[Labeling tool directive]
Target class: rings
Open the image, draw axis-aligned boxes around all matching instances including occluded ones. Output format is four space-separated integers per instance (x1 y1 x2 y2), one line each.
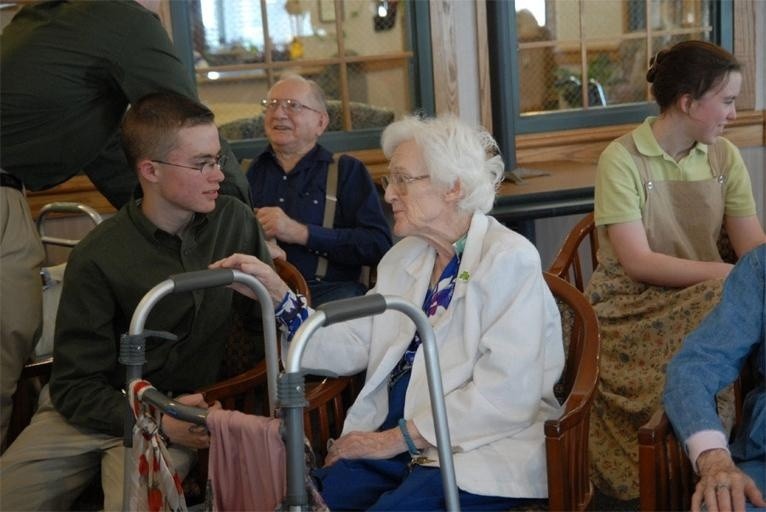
715 484 729 492
337 447 342 454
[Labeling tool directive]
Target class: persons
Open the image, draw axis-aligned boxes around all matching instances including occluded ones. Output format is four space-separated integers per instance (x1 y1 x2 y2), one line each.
244 72 393 310
0 90 277 512
206 111 566 511
579 37 764 512
0 0 257 462
663 240 766 512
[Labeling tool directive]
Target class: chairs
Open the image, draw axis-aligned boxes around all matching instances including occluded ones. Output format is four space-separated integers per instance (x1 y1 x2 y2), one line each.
166 260 312 510
546 213 739 293
304 269 600 512
641 341 758 511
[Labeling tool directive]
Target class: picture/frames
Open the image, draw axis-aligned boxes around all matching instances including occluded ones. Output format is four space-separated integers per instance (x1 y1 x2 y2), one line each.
319 2 347 25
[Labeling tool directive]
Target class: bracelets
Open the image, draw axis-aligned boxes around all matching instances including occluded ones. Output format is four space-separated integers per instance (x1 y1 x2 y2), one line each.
399 417 417 455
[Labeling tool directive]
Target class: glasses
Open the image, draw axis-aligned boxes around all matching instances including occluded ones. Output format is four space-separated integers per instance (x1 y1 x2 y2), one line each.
150 152 228 177
260 97 322 118
379 170 429 196
